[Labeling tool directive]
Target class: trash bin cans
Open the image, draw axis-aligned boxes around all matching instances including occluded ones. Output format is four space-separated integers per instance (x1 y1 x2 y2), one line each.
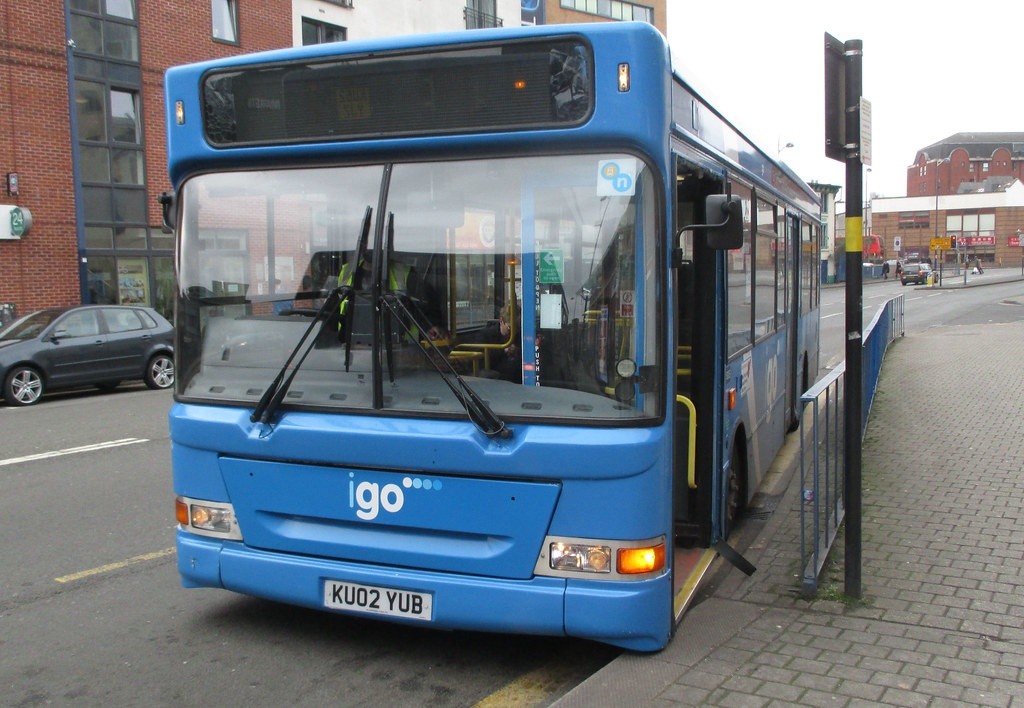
926 272 935 287
934 272 939 283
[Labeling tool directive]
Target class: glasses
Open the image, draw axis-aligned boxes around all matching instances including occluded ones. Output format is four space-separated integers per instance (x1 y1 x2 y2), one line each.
505 323 511 331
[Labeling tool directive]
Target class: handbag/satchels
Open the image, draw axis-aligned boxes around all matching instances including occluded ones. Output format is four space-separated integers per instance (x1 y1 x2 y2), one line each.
973 267 978 273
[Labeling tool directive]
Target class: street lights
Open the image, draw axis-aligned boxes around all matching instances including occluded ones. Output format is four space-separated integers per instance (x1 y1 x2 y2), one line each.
833 200 845 247
865 168 872 237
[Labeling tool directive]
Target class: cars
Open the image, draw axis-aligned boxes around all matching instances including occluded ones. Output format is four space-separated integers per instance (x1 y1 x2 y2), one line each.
0 306 178 408
900 263 939 286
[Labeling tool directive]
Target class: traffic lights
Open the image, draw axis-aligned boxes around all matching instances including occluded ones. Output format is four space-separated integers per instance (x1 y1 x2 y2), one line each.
951 235 957 249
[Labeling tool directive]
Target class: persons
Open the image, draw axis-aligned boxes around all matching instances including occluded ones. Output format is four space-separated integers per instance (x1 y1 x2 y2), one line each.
474 302 522 384
896 259 901 278
882 260 889 279
318 227 447 340
975 258 984 274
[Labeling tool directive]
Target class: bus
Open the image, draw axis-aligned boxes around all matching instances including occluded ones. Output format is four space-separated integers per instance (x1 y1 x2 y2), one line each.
158 22 828 656
835 235 884 260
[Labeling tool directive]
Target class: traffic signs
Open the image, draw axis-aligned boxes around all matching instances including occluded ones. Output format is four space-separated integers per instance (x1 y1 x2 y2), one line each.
539 249 567 284
